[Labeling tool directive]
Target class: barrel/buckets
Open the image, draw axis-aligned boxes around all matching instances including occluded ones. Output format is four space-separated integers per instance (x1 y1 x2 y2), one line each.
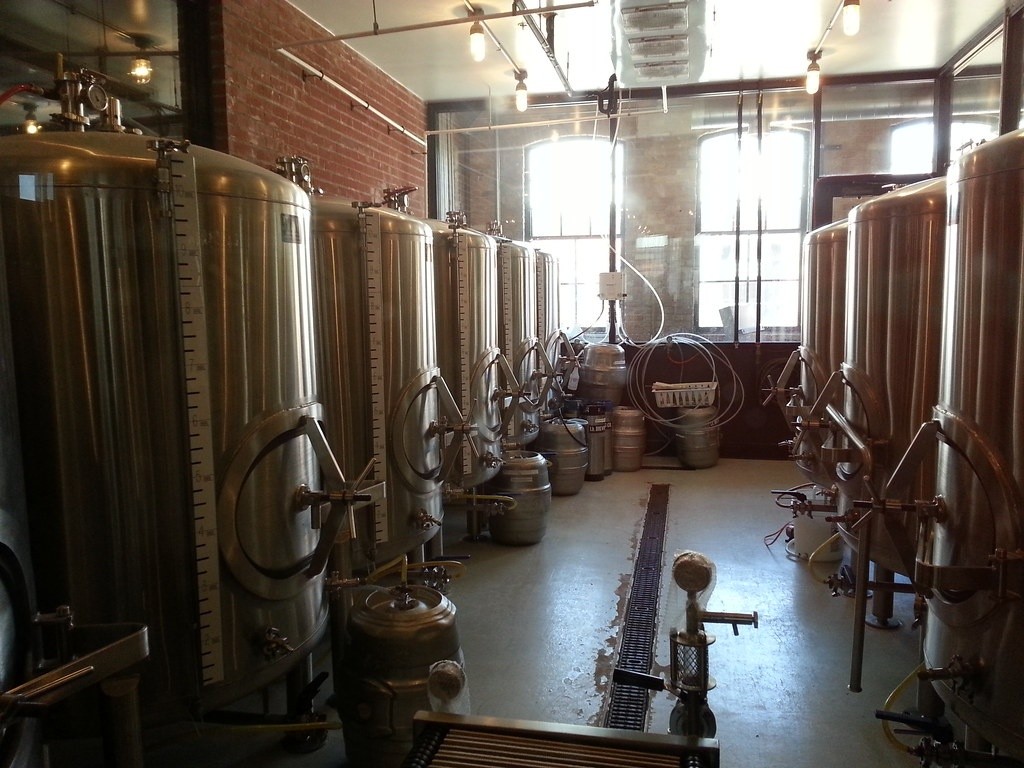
791 487 846 562
339 584 470 768
488 343 646 545
676 406 719 468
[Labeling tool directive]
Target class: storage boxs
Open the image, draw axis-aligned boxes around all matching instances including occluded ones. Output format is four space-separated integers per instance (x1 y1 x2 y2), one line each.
652 381 718 408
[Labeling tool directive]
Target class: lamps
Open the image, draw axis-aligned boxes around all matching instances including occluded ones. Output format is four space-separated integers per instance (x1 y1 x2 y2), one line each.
805 0 863 95
130 40 154 86
461 1 529 113
22 103 38 134
